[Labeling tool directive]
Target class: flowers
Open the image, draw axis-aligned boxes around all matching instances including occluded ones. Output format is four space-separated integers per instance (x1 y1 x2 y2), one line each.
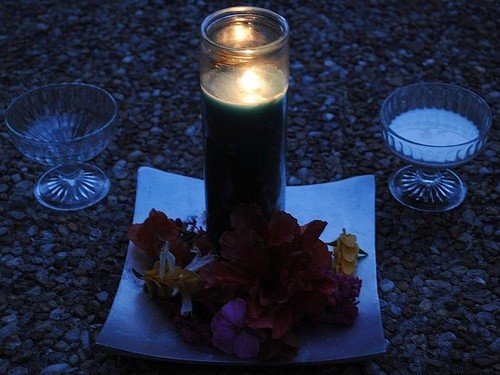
127 210 368 361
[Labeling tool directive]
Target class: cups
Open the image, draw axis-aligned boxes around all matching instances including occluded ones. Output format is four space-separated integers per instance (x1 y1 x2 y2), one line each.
198 7 291 253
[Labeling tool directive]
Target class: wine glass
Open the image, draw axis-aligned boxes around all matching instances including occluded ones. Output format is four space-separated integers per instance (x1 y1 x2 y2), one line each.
4 83 119 212
377 82 493 214
